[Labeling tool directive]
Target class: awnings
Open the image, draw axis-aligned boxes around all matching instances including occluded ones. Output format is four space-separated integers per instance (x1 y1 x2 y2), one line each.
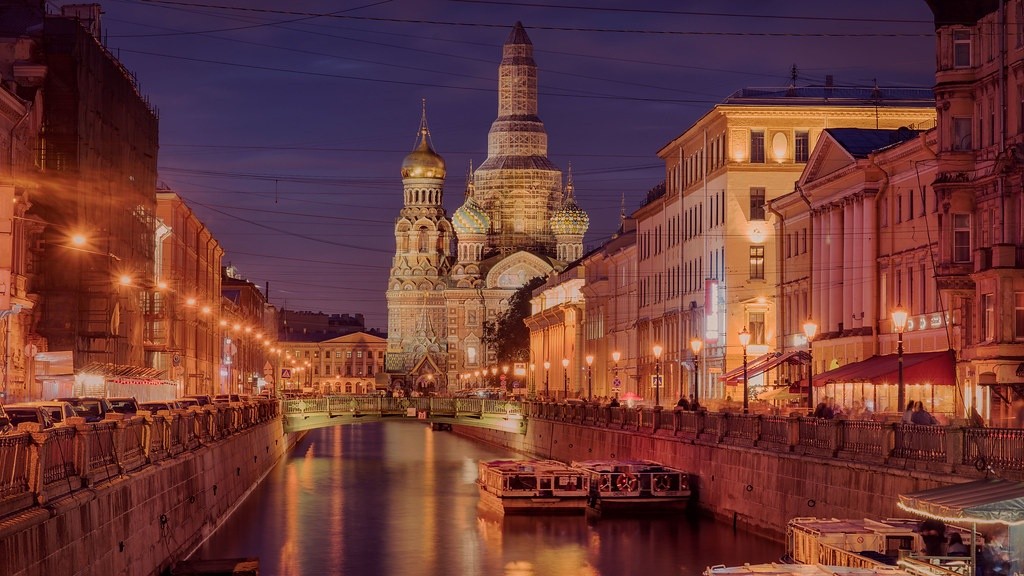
717 350 812 384
789 348 956 394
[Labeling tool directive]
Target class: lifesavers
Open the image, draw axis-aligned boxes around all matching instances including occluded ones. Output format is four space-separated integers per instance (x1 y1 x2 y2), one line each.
616 473 637 491
599 474 613 491
654 475 672 491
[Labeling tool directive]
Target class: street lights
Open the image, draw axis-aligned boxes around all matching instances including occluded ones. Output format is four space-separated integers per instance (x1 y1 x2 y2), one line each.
543 359 552 397
891 303 909 413
802 312 818 417
738 326 752 414
530 362 535 393
502 364 509 392
653 338 663 406
585 350 594 402
465 374 470 390
460 373 463 390
561 355 570 399
611 347 620 400
491 367 498 393
292 367 304 390
474 370 479 388
689 330 702 409
482 368 488 388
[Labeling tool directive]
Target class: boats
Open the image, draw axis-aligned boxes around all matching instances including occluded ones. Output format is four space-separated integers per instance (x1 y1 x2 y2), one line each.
779 478 1024 576
475 458 590 515
570 459 691 512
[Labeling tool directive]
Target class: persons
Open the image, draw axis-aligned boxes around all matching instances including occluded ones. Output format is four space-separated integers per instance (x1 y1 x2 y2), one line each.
911 401 938 426
982 535 1004 576
688 394 700 411
610 399 620 407
828 397 842 420
814 396 833 419
947 533 968 556
611 467 621 491
902 401 914 425
678 395 689 411
920 518 945 557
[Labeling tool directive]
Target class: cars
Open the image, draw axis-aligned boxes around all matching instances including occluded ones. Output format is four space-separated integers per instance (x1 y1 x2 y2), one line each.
0 390 323 432
562 398 584 418
